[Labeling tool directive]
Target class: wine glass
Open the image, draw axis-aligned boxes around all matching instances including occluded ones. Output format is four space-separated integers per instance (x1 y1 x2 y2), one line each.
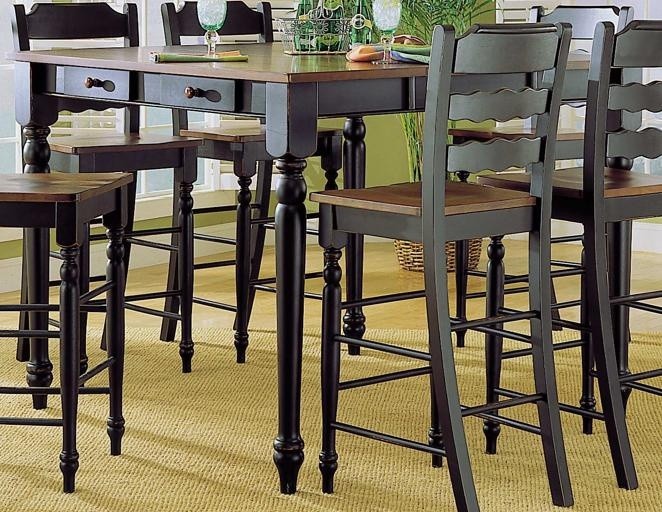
372 0 403 65
198 0 227 58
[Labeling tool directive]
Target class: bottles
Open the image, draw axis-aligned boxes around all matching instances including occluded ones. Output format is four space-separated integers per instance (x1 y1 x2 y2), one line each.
294 0 374 50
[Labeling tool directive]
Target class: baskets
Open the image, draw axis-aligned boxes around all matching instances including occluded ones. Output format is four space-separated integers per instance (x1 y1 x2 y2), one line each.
395 237 482 272
274 15 352 56
